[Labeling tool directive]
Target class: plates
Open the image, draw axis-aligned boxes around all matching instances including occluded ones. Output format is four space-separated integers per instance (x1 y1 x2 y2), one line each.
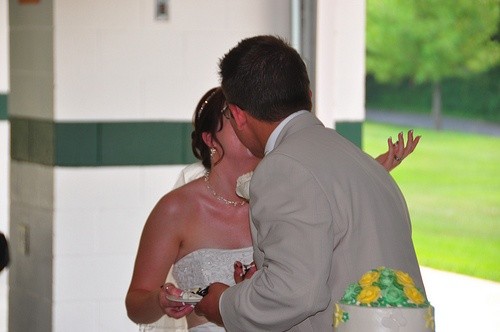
167 298 203 306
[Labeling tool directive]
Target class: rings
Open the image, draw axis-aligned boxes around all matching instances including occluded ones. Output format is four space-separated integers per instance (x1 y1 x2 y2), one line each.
394 156 402 161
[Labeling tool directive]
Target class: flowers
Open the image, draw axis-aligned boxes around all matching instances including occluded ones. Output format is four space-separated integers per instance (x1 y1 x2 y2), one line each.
236 171 254 199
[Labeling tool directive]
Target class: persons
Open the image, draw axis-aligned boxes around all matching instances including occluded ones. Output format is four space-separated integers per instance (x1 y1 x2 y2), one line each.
194 35 428 332
125 86 422 332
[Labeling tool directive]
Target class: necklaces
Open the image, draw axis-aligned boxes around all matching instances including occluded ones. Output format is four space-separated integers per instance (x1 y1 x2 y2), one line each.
203 171 249 208
